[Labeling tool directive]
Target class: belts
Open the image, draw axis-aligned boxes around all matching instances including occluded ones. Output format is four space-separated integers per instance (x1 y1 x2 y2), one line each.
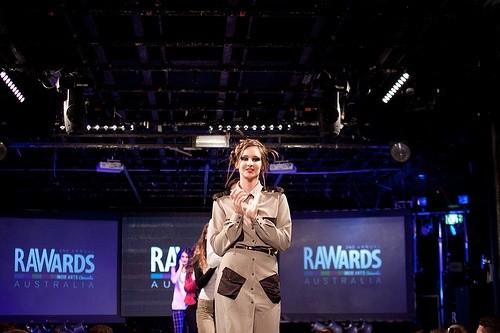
229 244 277 256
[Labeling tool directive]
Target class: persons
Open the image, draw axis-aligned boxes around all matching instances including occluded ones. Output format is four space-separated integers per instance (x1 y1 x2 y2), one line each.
210 140 291 333
170 223 214 333
